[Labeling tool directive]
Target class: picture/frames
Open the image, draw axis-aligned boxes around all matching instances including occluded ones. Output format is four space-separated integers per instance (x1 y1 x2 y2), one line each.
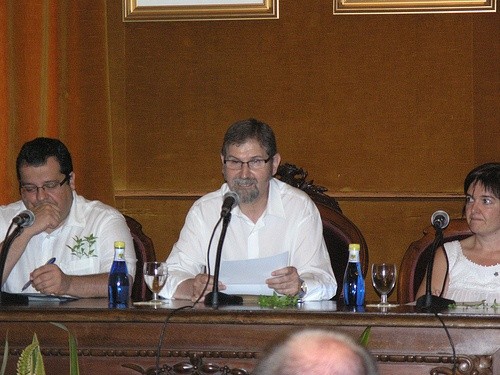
332 0 498 15
121 0 281 22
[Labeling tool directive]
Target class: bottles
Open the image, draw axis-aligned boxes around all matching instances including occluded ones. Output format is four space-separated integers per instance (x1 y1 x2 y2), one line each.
108 240 130 311
343 243 365 306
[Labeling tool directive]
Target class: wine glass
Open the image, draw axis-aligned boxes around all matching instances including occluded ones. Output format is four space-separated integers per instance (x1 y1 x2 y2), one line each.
143 262 168 304
371 263 397 304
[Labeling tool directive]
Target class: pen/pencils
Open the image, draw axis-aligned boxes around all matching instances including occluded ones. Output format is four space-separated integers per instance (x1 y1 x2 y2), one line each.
22 258 56 291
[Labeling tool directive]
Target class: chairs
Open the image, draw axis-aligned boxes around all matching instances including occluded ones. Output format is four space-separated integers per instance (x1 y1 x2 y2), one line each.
398 219 475 306
273 165 370 304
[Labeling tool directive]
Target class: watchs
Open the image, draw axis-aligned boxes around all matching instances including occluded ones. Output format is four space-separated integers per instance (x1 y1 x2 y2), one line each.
299 280 307 298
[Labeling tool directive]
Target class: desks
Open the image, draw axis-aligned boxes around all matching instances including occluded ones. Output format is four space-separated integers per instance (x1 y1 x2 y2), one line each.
0 294 500 375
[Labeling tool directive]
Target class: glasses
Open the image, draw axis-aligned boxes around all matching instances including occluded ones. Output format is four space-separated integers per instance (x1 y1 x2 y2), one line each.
223 153 274 170
18 175 69 195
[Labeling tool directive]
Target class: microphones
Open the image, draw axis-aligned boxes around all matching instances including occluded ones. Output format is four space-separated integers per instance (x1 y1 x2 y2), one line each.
12 210 35 227
219 191 240 216
431 210 450 233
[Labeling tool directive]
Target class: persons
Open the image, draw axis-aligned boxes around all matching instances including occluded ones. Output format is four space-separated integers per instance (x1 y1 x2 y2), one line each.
415 162 500 304
157 118 337 303
252 325 380 375
0 136 136 298
